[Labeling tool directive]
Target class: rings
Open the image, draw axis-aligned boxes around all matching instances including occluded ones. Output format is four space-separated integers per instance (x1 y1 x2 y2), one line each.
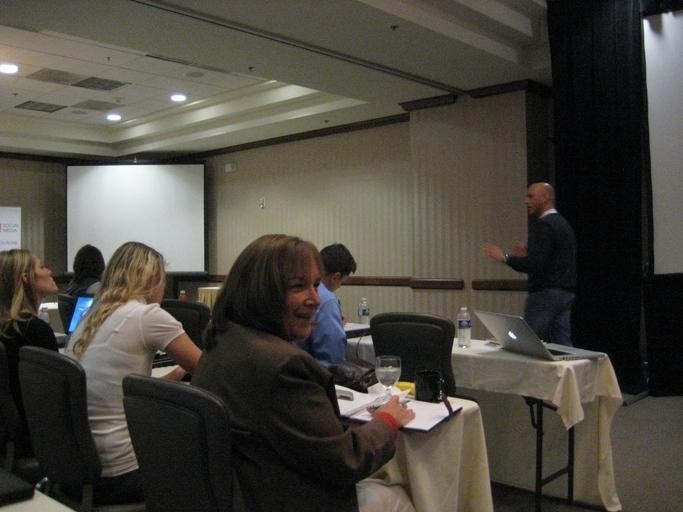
399 402 404 408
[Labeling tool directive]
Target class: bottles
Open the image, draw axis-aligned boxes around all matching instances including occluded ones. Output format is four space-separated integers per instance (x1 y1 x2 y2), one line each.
358 297 369 325
457 306 471 349
39 307 51 327
178 290 189 301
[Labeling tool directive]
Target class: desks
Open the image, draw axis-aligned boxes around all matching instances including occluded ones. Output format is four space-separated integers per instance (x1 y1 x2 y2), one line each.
345 314 624 511
198 285 222 313
330 384 495 512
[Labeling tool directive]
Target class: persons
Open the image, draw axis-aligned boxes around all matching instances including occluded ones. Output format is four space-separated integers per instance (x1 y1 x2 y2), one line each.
66 244 105 298
0 250 59 457
480 182 578 346
293 244 357 366
64 241 202 505
189 235 415 512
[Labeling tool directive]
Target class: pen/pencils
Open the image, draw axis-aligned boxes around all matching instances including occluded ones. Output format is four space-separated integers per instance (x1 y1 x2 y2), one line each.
366 399 410 413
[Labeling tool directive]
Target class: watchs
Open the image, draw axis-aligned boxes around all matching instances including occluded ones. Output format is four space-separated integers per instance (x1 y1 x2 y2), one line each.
503 249 512 265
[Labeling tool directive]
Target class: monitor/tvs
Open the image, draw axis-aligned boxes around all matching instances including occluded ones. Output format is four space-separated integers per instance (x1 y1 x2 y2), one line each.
65 294 95 336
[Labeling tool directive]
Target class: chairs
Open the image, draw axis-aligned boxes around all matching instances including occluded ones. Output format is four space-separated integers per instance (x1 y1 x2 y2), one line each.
57 294 78 334
370 312 456 402
122 374 243 512
160 300 210 345
18 345 102 510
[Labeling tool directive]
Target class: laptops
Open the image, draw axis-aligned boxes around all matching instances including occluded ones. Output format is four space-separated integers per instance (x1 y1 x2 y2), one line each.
473 308 604 362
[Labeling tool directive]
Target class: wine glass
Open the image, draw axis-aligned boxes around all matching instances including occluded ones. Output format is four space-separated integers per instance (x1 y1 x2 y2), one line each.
376 353 401 395
